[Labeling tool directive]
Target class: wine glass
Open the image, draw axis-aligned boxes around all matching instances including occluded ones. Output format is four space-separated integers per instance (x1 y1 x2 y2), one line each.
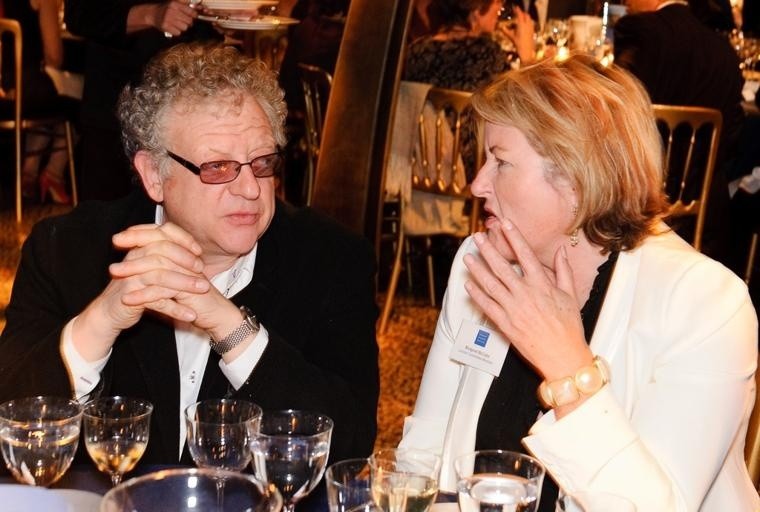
83 396 153 487
500 5 760 80
250 408 334 511
184 399 262 512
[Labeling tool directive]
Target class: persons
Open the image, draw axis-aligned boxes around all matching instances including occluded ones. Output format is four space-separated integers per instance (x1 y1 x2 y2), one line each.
1 43 380 512
612 0 745 240
1 0 70 205
63 0 247 190
402 1 536 221
398 55 758 512
500 0 599 42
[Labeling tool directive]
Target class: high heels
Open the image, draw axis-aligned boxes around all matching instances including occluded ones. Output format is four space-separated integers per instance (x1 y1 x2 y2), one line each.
40 172 69 205
21 173 36 202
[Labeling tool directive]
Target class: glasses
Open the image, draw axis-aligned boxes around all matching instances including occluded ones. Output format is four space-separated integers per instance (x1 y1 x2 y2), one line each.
164 147 285 185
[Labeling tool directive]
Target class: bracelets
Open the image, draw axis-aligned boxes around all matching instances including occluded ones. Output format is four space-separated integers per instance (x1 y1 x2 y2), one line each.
536 355 611 404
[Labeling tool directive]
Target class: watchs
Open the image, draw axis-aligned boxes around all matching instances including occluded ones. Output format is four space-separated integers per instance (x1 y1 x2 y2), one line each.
207 304 261 356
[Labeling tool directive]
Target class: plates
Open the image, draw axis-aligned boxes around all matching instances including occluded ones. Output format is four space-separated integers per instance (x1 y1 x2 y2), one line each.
195 12 299 31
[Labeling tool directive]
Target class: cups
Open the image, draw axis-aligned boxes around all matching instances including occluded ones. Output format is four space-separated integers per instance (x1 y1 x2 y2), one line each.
1 396 83 486
327 458 406 510
101 467 284 511
368 448 443 511
454 451 544 511
